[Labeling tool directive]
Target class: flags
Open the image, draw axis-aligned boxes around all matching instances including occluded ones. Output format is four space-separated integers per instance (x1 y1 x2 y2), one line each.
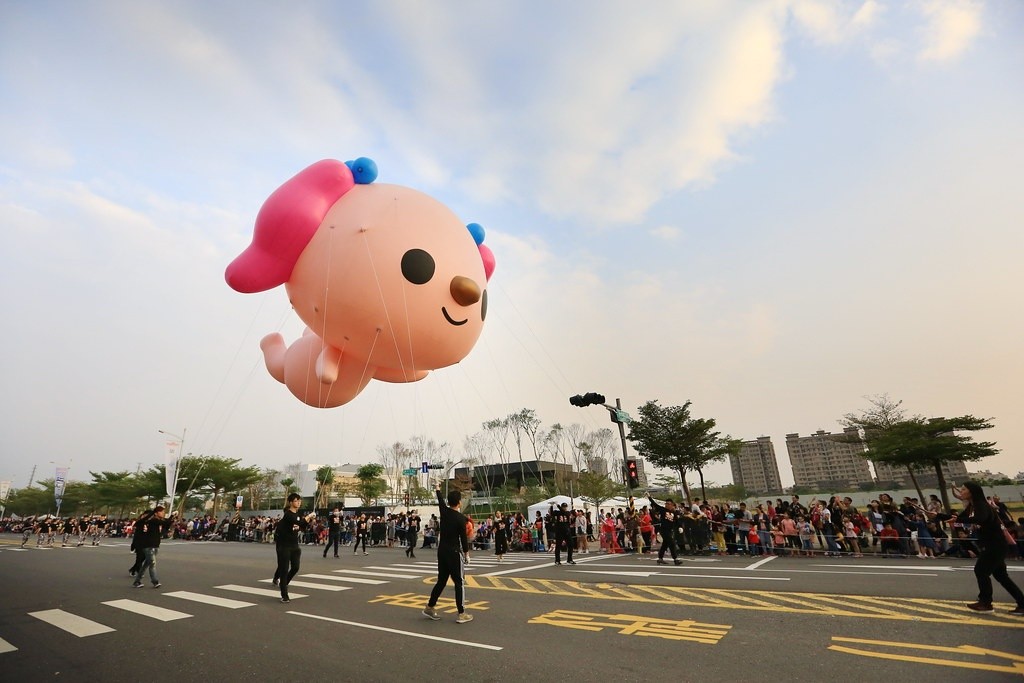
165 441 178 497
56 468 67 507
0 481 11 511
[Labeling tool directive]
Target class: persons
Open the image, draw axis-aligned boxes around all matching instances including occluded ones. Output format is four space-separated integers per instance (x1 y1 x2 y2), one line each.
133 507 178 588
422 477 473 623
322 507 343 558
273 493 316 603
956 481 1024 615
405 510 421 558
128 510 154 578
0 511 440 549
550 502 576 566
644 492 683 565
354 513 370 556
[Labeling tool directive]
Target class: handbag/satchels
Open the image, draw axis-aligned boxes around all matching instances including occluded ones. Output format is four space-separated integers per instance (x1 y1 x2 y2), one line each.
1001 524 1018 546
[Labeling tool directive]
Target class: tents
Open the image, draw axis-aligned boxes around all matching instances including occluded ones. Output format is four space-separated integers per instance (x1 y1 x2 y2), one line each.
528 495 595 532
599 496 665 519
465 484 1024 561
577 496 599 525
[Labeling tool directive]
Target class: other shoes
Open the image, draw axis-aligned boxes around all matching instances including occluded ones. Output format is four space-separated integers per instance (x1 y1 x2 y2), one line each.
323 551 326 557
555 561 561 566
132 581 144 588
282 597 290 603
405 550 409 557
674 560 683 565
273 579 280 586
567 560 577 564
364 552 369 556
677 552 972 560
354 551 358 556
577 549 616 555
497 556 504 562
657 559 669 565
129 570 137 577
334 554 339 557
154 583 163 589
411 554 416 558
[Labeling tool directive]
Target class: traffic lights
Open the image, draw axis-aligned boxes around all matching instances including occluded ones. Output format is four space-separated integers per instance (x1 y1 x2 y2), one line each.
570 394 585 408
584 392 605 405
627 459 640 488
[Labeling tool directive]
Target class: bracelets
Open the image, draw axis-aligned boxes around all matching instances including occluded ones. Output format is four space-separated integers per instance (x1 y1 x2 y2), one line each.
956 488 958 490
742 520 746 522
435 484 439 486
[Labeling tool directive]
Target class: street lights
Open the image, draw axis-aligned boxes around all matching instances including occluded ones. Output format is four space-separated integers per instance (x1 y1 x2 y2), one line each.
158 429 184 517
50 460 71 516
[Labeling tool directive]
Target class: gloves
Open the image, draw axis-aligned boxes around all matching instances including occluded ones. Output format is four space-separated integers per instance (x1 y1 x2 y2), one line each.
464 555 471 566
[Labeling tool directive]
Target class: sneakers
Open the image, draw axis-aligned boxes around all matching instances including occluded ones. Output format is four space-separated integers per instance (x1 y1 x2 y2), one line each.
966 601 995 613
456 613 474 623
422 608 441 621
1008 606 1024 616
